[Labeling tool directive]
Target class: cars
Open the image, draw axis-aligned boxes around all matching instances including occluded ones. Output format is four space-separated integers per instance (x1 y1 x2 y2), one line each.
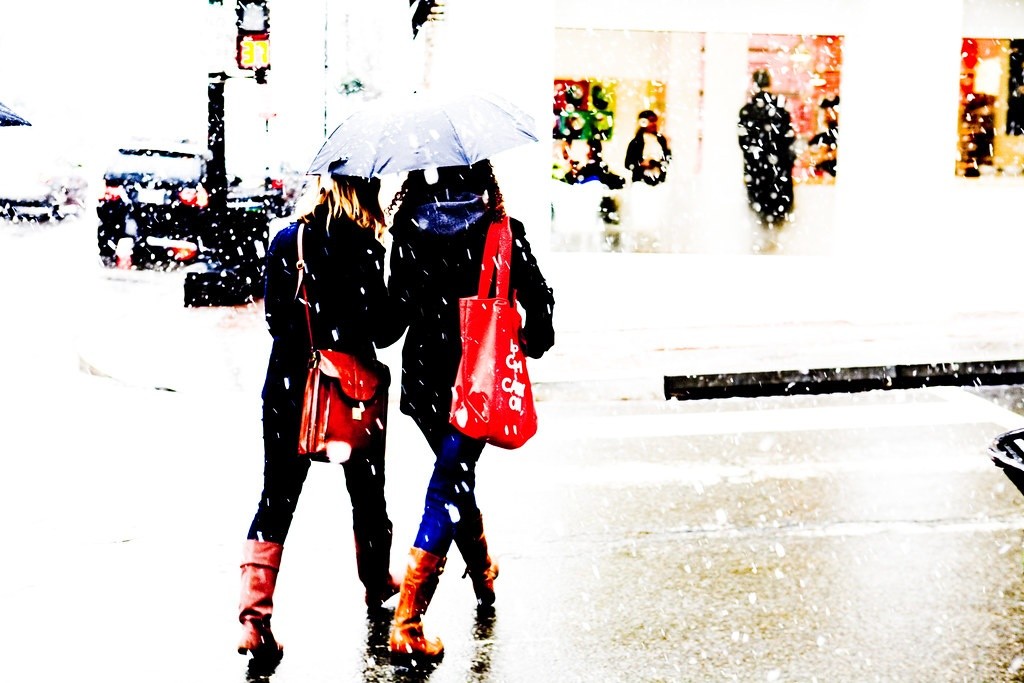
94 135 304 273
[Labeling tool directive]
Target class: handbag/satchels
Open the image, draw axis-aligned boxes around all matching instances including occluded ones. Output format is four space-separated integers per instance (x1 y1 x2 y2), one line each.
447 217 538 449
295 349 391 465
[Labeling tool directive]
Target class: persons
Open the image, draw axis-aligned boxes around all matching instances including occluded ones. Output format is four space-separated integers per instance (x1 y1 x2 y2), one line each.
802 94 841 185
959 71 996 178
625 110 671 187
737 68 797 253
555 132 627 247
384 158 556 656
234 172 403 660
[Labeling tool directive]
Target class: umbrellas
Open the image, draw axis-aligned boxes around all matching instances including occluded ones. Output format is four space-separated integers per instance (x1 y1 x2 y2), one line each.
305 86 540 184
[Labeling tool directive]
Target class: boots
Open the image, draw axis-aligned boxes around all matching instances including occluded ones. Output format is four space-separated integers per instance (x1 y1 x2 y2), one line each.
386 547 448 667
235 538 284 660
354 518 405 617
453 513 499 606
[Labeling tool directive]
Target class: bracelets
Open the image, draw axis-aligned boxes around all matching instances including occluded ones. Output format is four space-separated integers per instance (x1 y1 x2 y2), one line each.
827 151 833 159
